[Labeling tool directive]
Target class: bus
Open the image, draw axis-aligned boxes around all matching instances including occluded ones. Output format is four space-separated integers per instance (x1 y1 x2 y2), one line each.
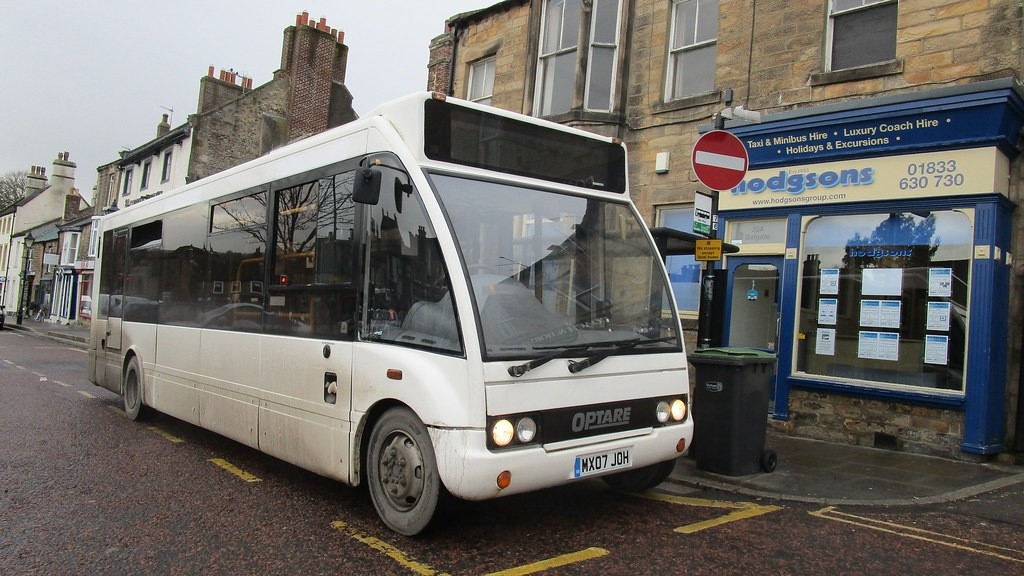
85 90 696 542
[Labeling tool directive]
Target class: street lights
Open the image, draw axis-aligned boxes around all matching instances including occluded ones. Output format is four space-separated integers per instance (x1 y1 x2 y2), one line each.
15 230 35 327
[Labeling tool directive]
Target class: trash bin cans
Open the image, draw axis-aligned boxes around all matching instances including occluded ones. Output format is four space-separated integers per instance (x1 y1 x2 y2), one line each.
686 346 777 476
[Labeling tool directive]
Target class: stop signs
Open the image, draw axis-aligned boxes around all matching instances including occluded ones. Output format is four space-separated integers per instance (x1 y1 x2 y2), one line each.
691 129 749 192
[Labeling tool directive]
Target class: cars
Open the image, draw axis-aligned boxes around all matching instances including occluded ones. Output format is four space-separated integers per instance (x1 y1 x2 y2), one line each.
99 293 315 338
80 295 92 319
